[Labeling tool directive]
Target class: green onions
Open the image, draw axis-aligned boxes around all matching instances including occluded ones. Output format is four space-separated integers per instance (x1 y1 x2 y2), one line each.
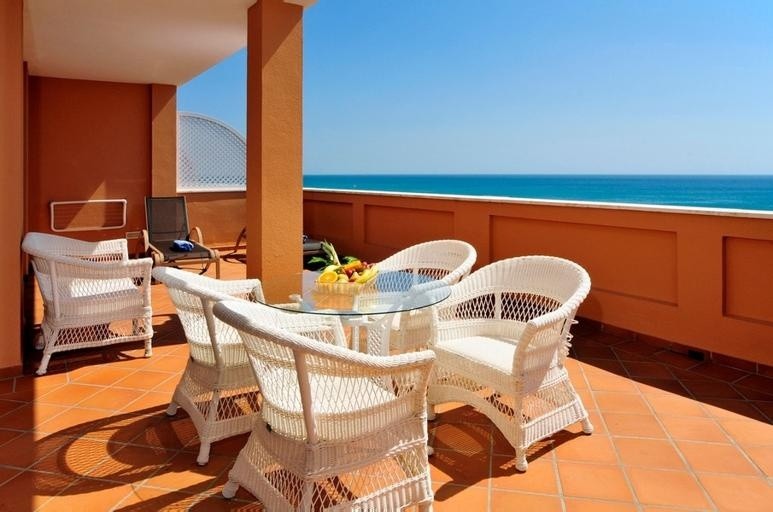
307 240 341 265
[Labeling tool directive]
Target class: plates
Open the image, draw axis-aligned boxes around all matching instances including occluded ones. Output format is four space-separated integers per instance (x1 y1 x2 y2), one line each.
313 271 379 296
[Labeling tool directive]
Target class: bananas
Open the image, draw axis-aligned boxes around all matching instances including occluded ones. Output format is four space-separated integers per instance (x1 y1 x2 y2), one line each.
353 268 376 283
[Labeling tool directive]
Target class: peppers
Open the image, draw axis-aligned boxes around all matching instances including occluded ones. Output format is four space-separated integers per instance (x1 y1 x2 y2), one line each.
342 256 358 264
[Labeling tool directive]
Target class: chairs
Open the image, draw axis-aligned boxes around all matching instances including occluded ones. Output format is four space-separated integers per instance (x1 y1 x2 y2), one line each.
352 239 478 350
21 232 155 377
233 225 325 269
422 254 594 473
151 266 265 468
141 195 221 284
213 297 434 512
